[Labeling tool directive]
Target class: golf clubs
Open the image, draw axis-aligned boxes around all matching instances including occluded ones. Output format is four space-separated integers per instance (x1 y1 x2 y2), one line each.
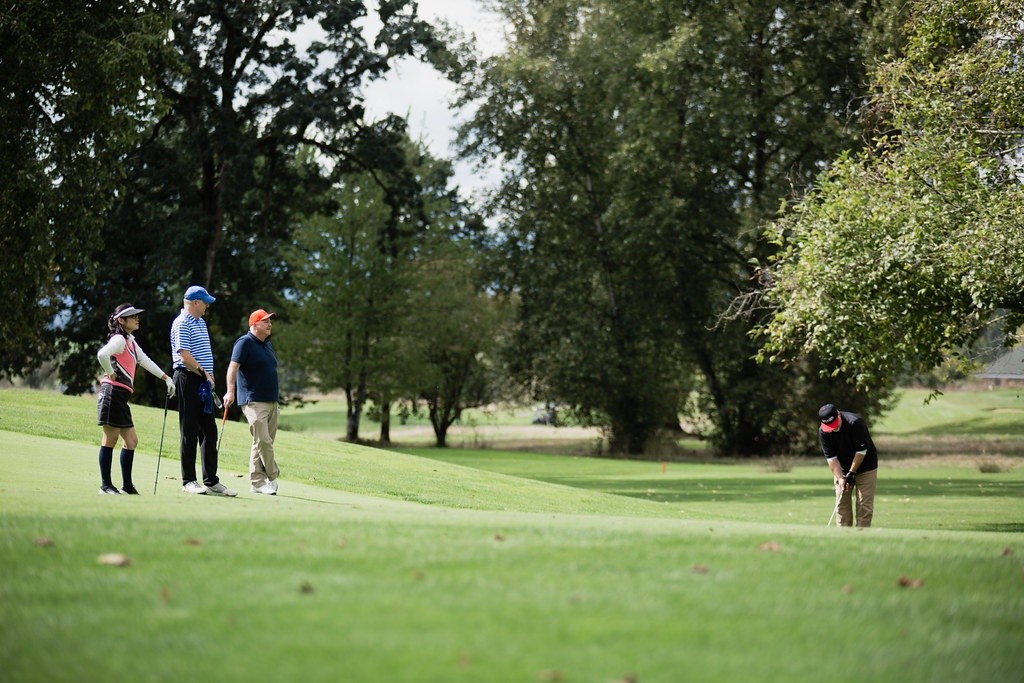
826 490 844 528
217 403 230 454
153 386 173 496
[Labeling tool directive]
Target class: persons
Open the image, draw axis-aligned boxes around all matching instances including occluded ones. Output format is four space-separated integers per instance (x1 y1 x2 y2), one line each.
223 309 281 496
170 286 239 497
97 304 176 496
819 404 878 527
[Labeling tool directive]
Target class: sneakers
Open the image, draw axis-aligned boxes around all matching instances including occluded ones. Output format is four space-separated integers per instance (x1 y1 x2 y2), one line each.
121 486 139 495
183 480 207 494
209 482 238 496
99 485 123 495
250 485 277 495
268 480 277 490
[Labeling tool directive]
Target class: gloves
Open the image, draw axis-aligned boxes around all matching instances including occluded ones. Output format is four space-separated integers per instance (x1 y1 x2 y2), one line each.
165 377 175 399
844 470 857 490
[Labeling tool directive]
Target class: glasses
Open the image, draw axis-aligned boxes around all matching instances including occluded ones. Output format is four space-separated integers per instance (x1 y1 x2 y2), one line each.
127 315 138 318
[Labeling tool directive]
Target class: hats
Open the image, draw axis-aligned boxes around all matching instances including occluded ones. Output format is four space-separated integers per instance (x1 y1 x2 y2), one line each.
819 404 839 432
249 309 277 326
113 307 144 320
184 286 216 303
